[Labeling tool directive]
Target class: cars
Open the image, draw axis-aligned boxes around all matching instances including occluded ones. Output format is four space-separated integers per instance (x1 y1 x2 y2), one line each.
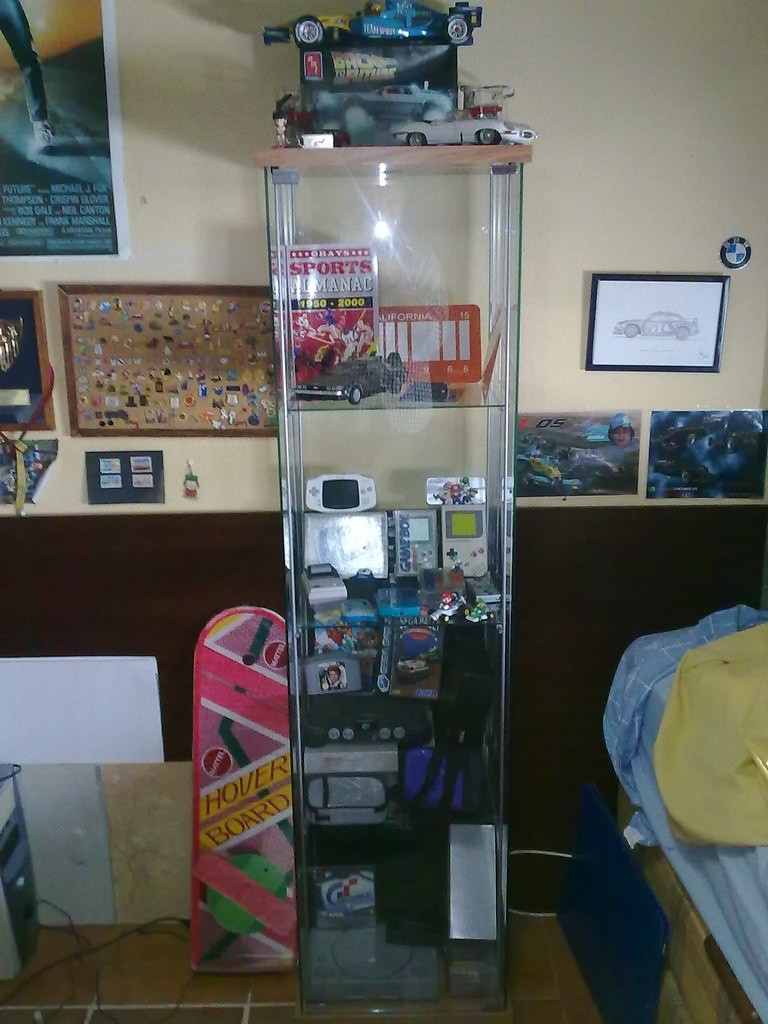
393 114 538 144
312 84 457 122
296 357 391 406
319 128 350 146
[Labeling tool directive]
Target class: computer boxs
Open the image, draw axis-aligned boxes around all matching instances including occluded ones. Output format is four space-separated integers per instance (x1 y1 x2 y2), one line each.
0 764 41 981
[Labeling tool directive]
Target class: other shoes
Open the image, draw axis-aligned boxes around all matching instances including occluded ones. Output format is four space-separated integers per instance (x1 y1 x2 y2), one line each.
32 121 53 152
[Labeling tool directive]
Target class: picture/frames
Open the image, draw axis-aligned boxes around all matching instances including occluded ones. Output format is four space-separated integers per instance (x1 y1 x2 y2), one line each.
585 273 731 373
0 289 56 431
56 283 278 436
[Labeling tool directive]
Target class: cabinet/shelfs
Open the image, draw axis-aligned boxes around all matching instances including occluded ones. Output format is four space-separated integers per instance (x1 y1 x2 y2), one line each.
256 147 532 1016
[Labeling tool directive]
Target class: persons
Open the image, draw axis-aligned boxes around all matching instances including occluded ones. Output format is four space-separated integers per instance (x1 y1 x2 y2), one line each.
604 413 641 468
327 665 344 691
0 0 56 147
